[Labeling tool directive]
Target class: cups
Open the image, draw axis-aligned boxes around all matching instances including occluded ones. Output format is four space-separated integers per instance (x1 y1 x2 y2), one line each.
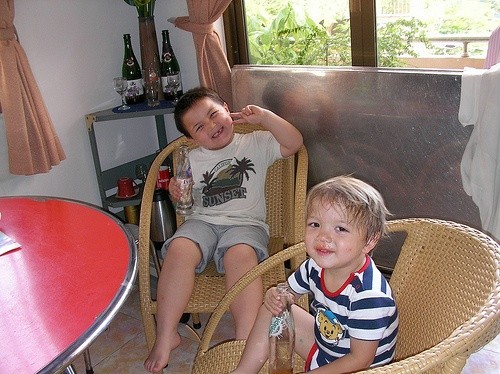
117 177 139 197
144 67 160 106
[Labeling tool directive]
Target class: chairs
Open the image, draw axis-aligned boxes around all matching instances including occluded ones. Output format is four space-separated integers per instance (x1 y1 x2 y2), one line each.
139 123 306 374
193 218 500 374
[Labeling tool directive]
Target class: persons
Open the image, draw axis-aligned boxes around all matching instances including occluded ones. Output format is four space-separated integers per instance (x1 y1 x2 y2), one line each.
144 87 303 374
227 175 398 374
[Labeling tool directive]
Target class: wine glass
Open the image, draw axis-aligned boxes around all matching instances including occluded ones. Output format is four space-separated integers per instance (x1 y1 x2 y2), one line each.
113 77 131 111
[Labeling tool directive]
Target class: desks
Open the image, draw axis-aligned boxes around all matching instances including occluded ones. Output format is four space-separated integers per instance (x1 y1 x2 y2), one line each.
0 195 138 374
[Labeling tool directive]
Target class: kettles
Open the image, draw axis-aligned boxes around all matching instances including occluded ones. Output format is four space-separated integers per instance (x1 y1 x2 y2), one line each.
149 186 177 249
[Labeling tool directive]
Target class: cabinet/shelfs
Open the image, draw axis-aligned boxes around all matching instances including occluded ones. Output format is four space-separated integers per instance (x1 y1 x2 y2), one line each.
85 102 174 212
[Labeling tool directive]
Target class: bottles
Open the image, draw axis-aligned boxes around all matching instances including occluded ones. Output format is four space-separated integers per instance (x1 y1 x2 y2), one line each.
268 282 296 374
120 34 145 105
158 29 183 101
175 144 195 215
